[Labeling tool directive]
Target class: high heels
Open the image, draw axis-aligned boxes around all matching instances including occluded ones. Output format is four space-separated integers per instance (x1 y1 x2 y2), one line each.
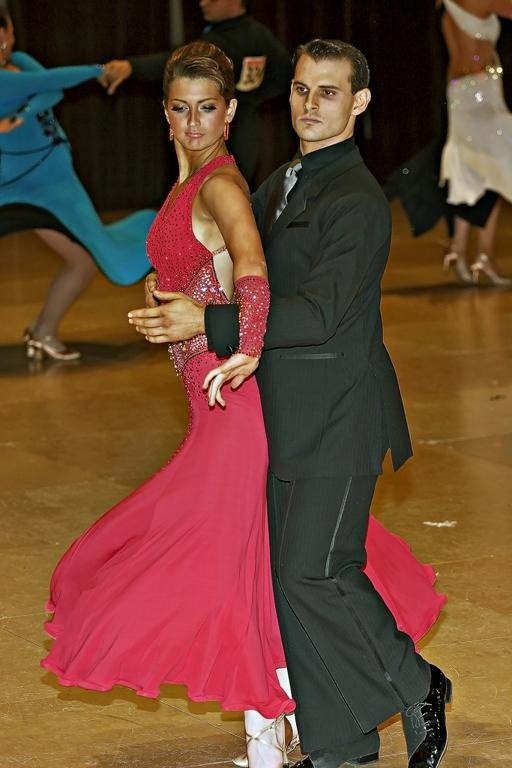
473 252 508 287
24 328 83 361
444 244 471 285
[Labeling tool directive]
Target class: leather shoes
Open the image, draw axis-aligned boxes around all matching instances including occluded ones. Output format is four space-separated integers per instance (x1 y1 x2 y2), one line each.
292 749 381 767
404 664 451 767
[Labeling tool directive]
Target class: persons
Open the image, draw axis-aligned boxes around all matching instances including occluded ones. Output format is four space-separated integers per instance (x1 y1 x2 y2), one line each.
96 0 293 194
0 0 160 362
38 39 451 767
439 1 511 288
124 36 451 768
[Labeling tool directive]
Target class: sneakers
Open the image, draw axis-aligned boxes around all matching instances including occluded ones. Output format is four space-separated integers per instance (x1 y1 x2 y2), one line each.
230 750 249 768
